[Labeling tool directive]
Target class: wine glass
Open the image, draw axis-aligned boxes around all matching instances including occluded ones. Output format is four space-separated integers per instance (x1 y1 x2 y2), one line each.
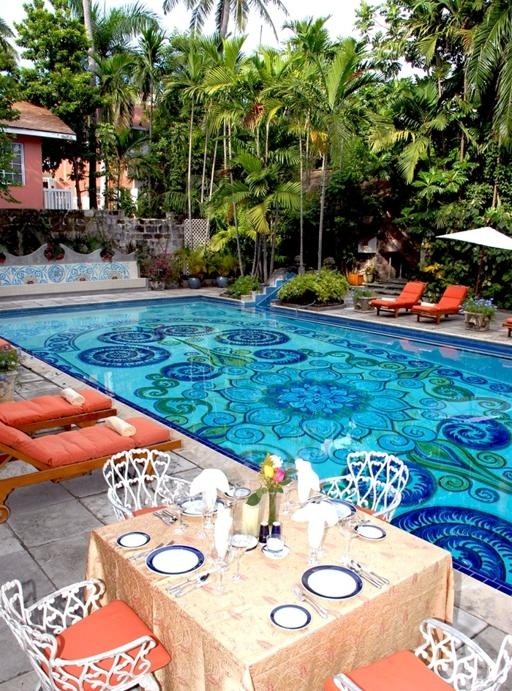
203 492 215 529
226 525 251 582
171 489 188 533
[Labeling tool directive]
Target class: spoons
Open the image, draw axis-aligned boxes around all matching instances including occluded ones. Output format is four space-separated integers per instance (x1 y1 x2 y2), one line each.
351 560 383 588
173 572 211 599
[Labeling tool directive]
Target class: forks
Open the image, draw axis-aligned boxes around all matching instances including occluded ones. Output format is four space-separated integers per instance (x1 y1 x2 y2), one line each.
168 570 211 591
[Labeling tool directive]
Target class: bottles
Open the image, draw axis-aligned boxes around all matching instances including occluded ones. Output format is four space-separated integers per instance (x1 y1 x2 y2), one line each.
271 522 281 541
258 522 269 544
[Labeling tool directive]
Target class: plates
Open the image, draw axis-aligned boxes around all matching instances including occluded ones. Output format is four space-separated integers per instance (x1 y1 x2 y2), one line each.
354 523 388 542
116 531 152 550
317 498 357 527
223 487 251 500
260 543 290 560
300 564 364 602
178 496 225 517
268 603 313 631
144 544 206 578
229 534 258 552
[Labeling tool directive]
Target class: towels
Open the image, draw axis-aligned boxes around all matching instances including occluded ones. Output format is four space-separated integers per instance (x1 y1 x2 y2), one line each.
104 415 136 438
295 458 321 502
213 502 234 559
189 468 231 512
61 387 85 407
292 502 339 549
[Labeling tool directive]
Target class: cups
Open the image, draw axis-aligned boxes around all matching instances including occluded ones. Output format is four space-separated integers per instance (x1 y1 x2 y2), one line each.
266 534 286 554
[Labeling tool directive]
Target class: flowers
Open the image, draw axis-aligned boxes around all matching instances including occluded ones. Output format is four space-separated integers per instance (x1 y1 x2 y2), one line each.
463 295 496 325
0 344 22 374
150 259 173 281
352 287 374 304
245 451 298 525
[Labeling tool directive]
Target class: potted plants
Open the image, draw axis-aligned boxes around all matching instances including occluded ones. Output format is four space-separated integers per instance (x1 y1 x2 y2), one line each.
172 244 207 289
205 253 237 288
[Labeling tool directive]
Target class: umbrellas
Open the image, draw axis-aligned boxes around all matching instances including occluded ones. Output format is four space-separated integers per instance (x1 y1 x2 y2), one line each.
436 226 512 300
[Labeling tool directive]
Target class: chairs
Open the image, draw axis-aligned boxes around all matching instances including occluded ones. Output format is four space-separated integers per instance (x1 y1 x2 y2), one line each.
412 285 468 325
317 451 410 525
0 579 171 691
0 388 117 469
371 281 425 318
0 416 182 524
324 617 512 691
102 449 192 521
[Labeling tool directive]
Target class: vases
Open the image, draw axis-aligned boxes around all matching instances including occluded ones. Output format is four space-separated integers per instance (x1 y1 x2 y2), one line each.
464 310 491 332
0 370 18 403
149 280 166 291
269 493 278 527
354 297 376 313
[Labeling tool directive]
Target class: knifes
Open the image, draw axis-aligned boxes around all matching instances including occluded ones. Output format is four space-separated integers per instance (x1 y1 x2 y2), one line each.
347 564 382 590
